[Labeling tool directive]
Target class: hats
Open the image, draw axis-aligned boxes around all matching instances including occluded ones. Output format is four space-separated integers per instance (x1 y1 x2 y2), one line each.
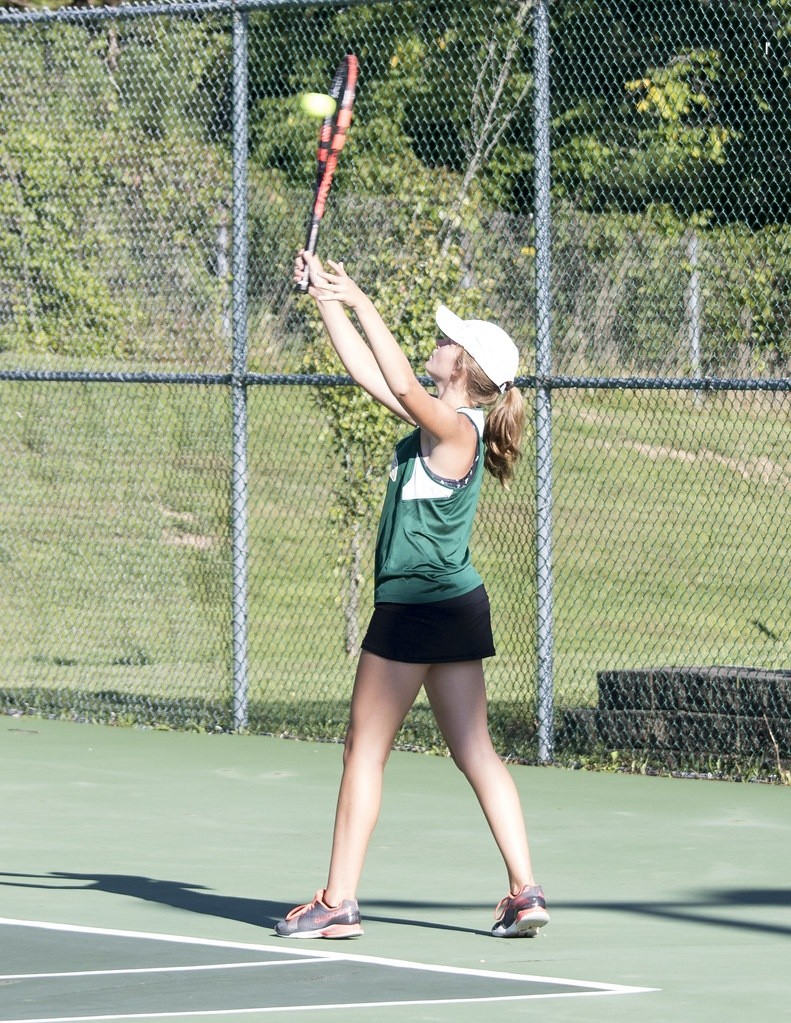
435 304 520 394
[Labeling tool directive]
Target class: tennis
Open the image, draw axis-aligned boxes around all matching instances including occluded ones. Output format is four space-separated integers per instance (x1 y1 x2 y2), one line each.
298 90 336 119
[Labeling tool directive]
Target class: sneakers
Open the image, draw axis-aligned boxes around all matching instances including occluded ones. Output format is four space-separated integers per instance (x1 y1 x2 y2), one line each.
492 885 548 938
275 889 363 939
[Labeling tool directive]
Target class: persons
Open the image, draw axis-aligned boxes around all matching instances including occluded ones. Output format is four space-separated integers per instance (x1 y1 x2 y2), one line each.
275 249 547 936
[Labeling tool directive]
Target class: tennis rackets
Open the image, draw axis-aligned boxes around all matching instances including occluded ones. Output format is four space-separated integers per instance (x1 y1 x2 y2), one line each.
292 54 358 293
